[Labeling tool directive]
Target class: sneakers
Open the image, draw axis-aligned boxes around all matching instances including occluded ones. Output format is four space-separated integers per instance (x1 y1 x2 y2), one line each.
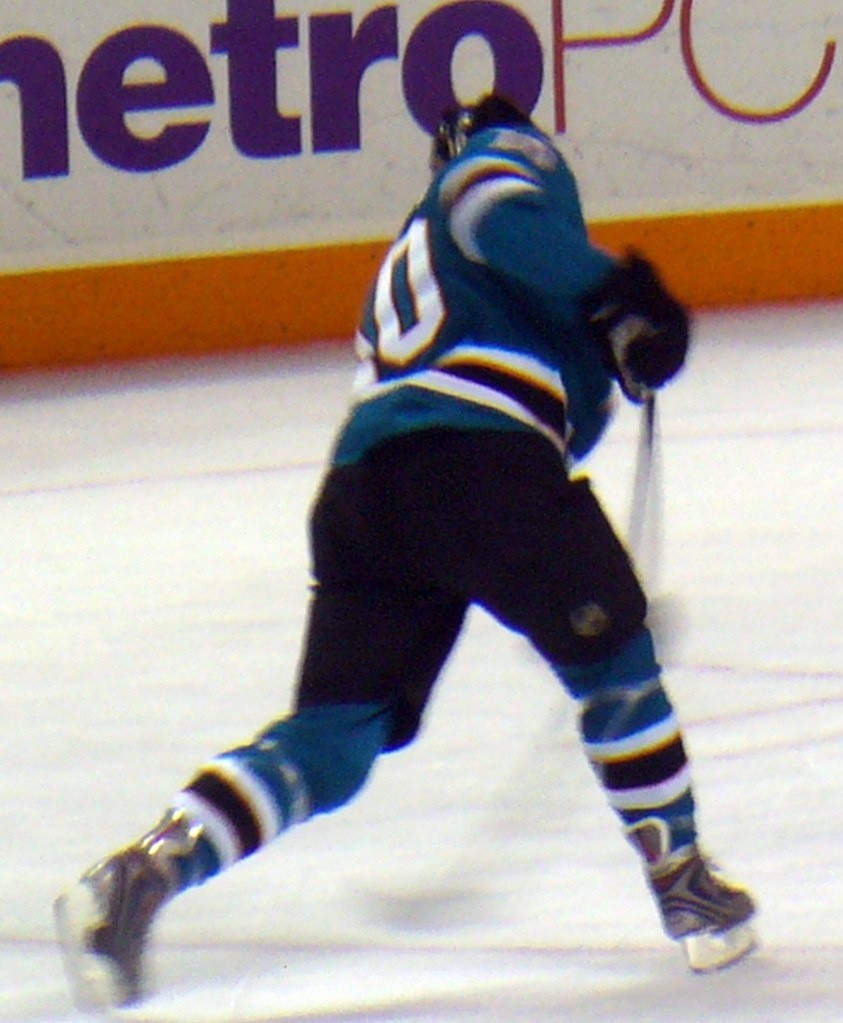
626 819 758 971
50 803 201 1016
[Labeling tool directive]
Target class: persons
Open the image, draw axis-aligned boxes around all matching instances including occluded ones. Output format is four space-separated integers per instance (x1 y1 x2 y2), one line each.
80 94 757 1002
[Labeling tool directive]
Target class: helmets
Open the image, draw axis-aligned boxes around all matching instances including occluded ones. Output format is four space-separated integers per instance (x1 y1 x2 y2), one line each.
426 94 531 175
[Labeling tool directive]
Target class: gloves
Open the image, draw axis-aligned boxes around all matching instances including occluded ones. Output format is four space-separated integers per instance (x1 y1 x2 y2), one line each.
578 254 689 402
